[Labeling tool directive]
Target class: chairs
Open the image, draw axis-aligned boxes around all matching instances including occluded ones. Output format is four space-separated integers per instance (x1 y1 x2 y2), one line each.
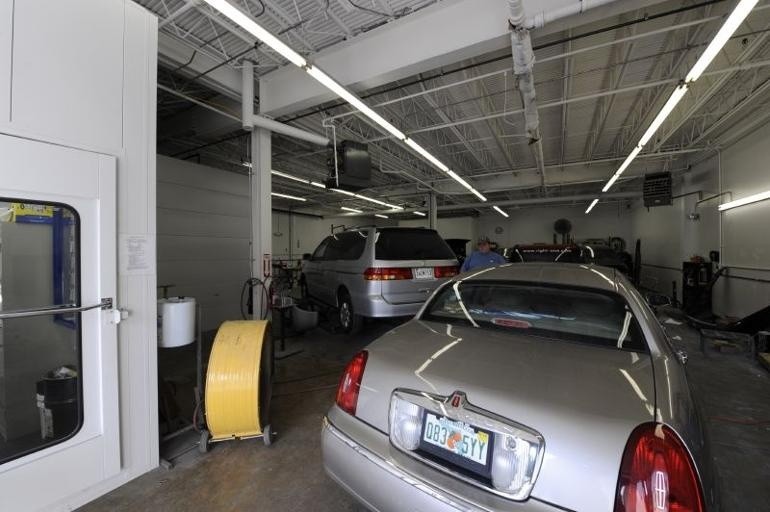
483 290 622 327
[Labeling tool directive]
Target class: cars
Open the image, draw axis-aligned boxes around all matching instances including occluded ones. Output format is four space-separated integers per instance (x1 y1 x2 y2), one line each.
319 261 719 511
299 226 459 336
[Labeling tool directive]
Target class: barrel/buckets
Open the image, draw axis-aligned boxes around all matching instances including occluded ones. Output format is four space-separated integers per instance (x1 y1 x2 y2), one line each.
33 366 78 442
157 294 196 350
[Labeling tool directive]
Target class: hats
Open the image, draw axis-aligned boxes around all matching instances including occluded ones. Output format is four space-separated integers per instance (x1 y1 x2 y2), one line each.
478 235 489 244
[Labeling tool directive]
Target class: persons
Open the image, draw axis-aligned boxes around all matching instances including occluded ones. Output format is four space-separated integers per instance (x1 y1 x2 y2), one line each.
459 233 507 275
